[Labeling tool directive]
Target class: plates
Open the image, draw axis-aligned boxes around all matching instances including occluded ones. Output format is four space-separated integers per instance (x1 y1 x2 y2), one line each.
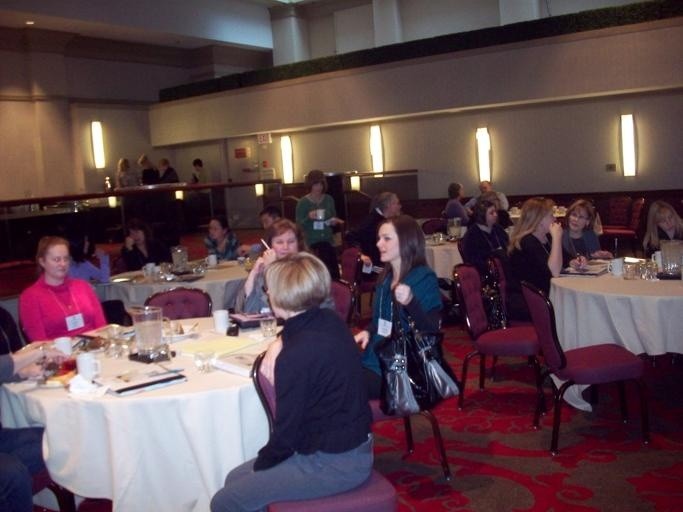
109 274 133 283
159 323 202 339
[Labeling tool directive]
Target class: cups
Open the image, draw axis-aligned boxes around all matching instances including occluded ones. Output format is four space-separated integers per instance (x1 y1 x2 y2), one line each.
143 262 155 273
260 319 277 342
159 263 170 275
53 336 73 358
316 209 326 221
205 254 218 268
194 338 218 374
607 250 661 281
77 352 102 381
213 310 231 332
431 234 443 247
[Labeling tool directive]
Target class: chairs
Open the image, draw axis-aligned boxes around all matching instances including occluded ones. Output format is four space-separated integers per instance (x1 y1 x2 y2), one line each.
598 193 645 259
251 350 400 512
331 245 377 328
452 238 651 456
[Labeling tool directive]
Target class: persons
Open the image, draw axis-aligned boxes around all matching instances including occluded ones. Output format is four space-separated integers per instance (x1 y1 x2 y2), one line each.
119 218 161 270
444 181 512 280
233 218 334 315
18 236 107 342
205 205 282 261
0 338 67 512
295 169 344 282
505 196 615 295
67 228 110 284
116 152 204 187
358 192 401 279
353 215 442 402
642 202 683 260
208 250 375 511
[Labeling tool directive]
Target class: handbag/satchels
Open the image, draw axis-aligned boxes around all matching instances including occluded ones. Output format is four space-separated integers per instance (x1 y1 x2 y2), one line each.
481 282 507 330
228 312 284 328
375 332 462 417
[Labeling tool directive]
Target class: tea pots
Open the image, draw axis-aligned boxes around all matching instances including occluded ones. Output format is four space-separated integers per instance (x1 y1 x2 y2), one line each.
447 217 463 239
659 240 683 276
132 304 166 356
171 246 189 273
